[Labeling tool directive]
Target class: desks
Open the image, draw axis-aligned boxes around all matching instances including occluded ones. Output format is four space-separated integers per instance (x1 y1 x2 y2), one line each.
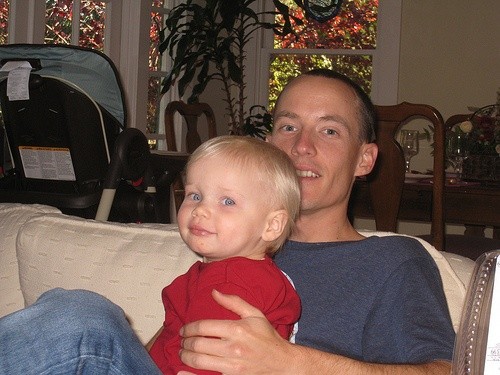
347 175 500 238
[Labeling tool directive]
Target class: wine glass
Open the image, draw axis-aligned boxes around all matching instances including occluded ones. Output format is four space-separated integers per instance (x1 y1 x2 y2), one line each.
399 130 419 173
446 131 471 183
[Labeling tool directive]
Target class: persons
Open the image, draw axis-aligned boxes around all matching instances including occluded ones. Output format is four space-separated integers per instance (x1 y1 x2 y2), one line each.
147 135 301 374
0 69 454 375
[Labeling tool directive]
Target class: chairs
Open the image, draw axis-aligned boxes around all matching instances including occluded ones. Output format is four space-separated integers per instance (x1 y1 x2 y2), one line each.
165 102 217 189
347 101 500 262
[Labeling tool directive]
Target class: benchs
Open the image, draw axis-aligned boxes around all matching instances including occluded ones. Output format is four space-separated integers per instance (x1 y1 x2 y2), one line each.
0 202 500 375
